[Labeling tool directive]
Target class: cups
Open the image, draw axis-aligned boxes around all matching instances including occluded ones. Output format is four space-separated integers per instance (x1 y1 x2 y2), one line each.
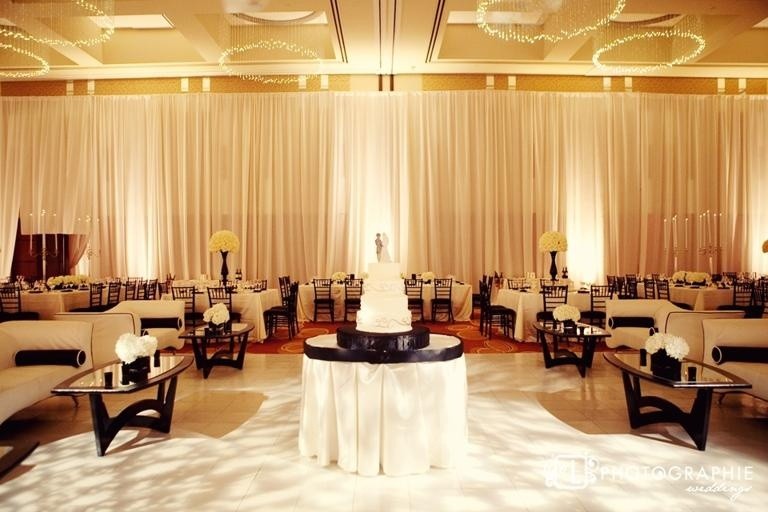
579 326 584 335
16 276 25 282
204 327 209 336
639 348 647 367
104 372 113 389
688 367 697 382
154 350 161 367
553 320 557 329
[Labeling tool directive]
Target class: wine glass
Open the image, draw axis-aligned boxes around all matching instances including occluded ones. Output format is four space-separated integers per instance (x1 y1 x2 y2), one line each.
233 278 262 292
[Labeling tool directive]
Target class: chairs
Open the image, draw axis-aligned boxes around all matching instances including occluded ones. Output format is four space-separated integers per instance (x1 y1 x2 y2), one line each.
0 267 301 343
474 263 768 342
290 268 472 326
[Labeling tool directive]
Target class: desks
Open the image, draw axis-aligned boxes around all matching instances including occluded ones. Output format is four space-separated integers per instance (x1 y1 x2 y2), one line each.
602 348 753 452
49 353 195 456
533 321 613 378
178 322 255 379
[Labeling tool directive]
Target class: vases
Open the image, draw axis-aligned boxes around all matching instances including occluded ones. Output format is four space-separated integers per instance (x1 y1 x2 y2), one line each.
219 247 230 288
549 249 559 283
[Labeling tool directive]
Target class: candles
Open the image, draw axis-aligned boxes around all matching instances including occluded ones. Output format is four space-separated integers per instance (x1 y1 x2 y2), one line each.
695 209 729 256
662 213 690 257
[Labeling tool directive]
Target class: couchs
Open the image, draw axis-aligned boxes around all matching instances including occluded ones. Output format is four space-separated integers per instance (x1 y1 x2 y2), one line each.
700 316 768 421
54 299 188 369
602 296 745 365
0 319 97 429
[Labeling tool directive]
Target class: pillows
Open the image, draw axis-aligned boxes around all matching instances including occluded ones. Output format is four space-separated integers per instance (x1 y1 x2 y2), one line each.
12 348 87 367
712 344 768 365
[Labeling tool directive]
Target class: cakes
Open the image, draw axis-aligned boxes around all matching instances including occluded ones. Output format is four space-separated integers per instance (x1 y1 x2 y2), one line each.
356 233 411 330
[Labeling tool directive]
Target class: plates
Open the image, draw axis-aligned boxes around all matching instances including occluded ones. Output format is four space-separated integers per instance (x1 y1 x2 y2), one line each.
80 288 88 290
540 290 551 294
717 286 729 289
675 285 683 287
690 286 699 288
578 291 589 293
29 290 43 293
61 290 73 292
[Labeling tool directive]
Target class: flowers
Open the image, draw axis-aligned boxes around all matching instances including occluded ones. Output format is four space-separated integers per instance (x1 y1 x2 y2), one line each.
537 230 568 254
116 332 158 362
205 229 242 256
643 332 690 363
